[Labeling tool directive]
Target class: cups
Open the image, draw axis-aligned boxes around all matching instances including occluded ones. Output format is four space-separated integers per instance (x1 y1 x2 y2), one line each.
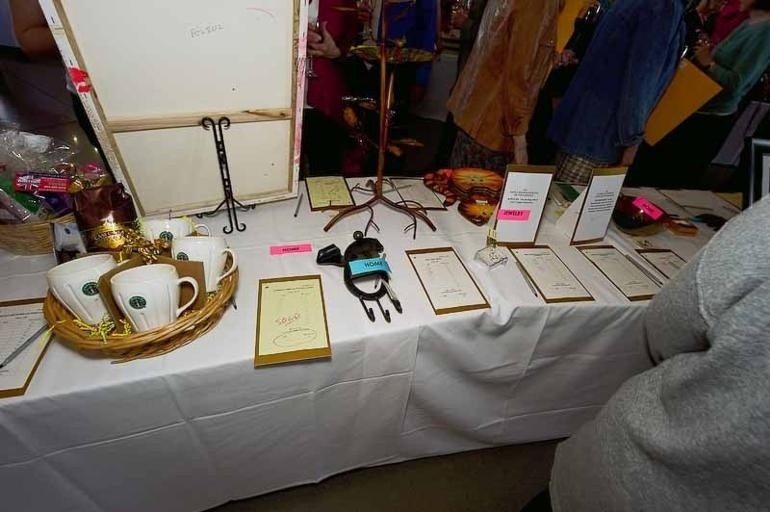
141 218 212 242
46 253 131 326
171 236 237 293
109 263 198 332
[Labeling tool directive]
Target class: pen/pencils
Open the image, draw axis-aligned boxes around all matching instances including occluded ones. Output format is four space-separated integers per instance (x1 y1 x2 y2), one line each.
683 205 714 211
0 323 49 369
516 262 537 297
626 254 663 285
375 253 386 289
383 184 412 193
231 295 237 310
294 192 303 217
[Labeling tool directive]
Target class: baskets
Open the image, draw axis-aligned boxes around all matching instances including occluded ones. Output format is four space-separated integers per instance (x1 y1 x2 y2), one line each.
0 213 77 255
40 231 242 366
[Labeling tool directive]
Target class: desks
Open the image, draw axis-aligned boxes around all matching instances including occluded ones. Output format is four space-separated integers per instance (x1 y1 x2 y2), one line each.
0 173 742 511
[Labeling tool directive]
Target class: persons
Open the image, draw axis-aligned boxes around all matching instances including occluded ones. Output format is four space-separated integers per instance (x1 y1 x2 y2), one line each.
519 194 769 512
299 0 770 190
8 0 117 183
